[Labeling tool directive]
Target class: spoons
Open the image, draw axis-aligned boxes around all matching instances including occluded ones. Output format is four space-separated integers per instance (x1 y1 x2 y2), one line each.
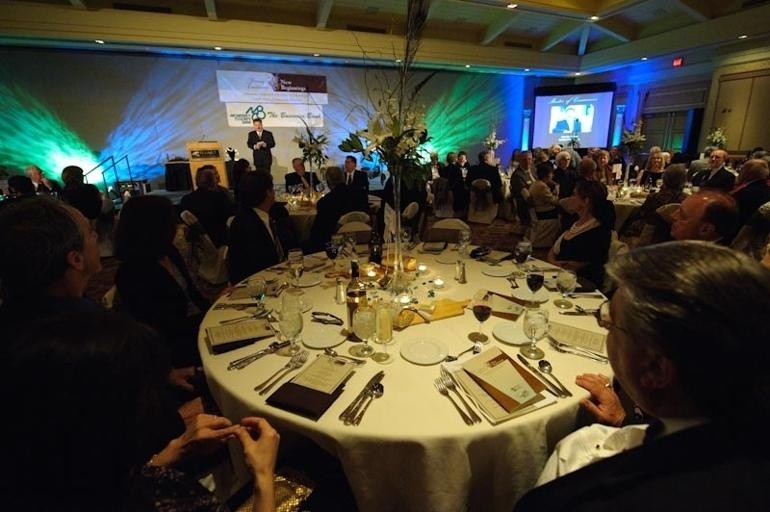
355 384 383 428
537 359 571 397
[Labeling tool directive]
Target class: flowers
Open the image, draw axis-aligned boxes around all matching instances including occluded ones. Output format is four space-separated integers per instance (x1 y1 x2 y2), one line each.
704 127 729 154
618 120 648 181
483 129 506 150
323 0 443 271
294 116 329 196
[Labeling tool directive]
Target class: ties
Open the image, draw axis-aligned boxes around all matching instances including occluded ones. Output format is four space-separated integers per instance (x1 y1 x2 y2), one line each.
269 214 287 264
699 168 714 187
348 171 352 186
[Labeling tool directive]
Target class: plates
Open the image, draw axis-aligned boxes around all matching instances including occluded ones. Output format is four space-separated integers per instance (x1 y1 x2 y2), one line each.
400 337 448 365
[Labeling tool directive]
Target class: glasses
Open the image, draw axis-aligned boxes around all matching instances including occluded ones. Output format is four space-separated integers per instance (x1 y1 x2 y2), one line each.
597 299 664 360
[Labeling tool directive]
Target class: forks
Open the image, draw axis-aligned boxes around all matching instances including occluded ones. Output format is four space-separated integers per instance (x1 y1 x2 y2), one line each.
433 372 478 426
547 334 609 363
254 350 309 395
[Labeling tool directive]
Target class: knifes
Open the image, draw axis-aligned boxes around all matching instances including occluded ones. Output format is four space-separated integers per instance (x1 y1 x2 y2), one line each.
340 370 385 425
559 311 608 316
229 342 277 372
517 353 563 397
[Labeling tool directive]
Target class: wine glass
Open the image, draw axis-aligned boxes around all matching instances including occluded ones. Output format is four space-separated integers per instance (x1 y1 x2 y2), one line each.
247 275 269 317
279 228 576 360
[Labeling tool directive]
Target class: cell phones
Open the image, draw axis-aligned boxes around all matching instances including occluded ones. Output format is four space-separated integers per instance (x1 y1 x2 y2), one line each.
472 246 490 256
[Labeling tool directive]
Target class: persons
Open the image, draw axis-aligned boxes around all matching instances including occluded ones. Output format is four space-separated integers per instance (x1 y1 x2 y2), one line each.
248 116 276 173
1 148 770 510
552 109 584 133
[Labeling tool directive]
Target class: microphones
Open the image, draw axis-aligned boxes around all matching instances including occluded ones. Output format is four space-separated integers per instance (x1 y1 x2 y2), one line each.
198 134 206 143
